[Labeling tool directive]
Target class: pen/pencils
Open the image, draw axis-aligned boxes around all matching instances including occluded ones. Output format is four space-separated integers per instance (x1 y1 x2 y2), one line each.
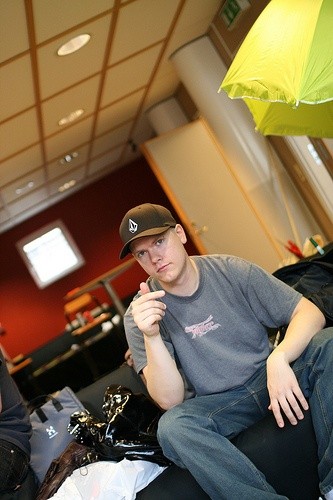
309 237 325 255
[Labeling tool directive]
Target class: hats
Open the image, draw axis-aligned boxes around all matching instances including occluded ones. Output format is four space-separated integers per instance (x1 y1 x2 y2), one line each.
119 203 176 260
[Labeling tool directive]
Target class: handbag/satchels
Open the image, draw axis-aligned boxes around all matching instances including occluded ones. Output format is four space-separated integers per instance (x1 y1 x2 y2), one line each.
29 386 85 485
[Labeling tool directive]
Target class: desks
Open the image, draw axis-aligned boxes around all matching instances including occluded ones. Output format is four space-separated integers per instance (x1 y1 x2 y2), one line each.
66 258 136 319
9 359 43 406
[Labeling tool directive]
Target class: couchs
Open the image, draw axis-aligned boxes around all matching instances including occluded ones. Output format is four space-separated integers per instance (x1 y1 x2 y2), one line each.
76 364 319 499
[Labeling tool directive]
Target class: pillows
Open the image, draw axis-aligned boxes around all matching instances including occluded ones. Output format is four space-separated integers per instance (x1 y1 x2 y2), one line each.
47 460 167 499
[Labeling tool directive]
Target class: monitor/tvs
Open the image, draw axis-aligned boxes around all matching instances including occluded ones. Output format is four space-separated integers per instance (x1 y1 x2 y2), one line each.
15 219 87 290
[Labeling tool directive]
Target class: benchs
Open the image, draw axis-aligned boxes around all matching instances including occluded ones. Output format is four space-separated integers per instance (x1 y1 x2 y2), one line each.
27 291 137 402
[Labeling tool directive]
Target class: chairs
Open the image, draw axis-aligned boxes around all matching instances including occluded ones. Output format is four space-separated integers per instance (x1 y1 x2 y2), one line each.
64 294 127 367
